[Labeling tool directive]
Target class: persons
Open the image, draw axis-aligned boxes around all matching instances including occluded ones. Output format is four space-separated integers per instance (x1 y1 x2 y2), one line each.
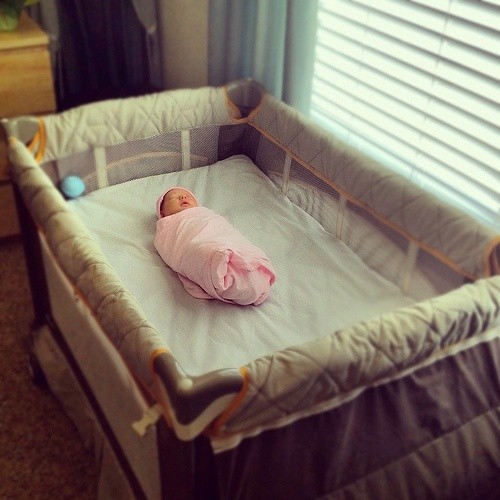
153 185 279 308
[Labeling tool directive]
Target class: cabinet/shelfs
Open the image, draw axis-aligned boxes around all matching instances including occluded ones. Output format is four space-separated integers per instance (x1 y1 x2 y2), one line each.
1 7 56 236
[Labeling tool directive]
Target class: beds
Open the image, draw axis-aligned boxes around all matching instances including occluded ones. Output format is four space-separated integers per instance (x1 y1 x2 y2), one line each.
1 75 500 500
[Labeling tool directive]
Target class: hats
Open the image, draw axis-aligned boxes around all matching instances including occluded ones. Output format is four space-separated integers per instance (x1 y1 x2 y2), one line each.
156 186 199 220
